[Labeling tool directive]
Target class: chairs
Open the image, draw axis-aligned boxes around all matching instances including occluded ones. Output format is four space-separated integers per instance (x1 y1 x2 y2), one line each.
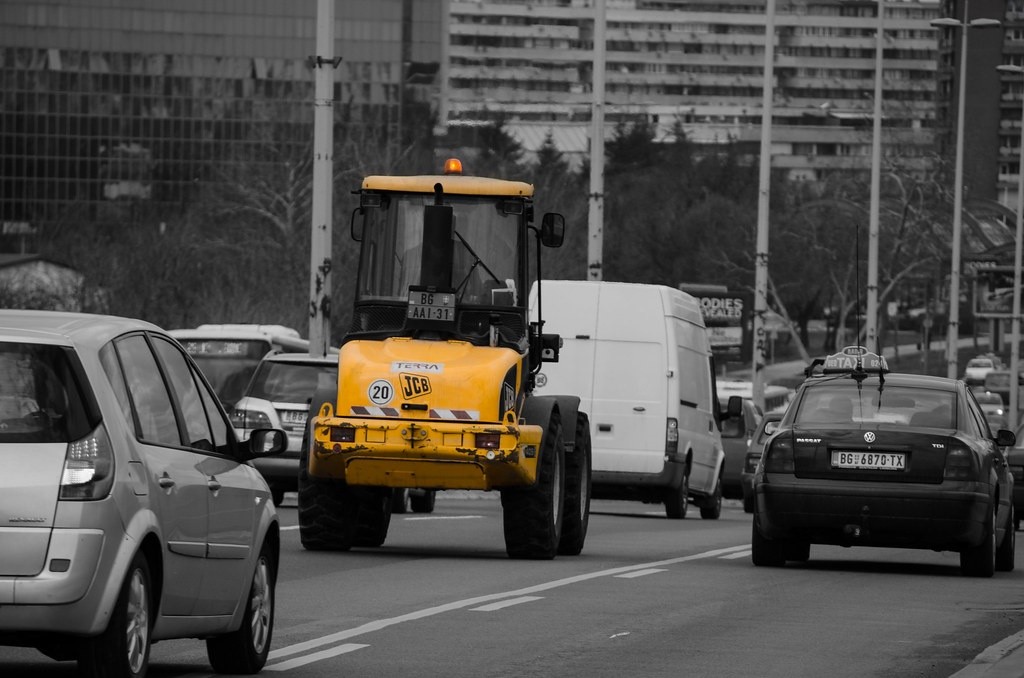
273 369 320 405
808 409 853 425
909 411 951 428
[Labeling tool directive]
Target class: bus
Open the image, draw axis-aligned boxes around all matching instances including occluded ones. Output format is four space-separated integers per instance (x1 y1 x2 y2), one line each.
160 322 344 414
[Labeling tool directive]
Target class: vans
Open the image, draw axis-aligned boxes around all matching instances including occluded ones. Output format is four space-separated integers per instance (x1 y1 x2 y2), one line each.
524 278 744 520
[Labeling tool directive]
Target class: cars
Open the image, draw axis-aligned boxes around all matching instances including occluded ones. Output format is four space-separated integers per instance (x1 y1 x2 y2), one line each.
1 312 291 678
713 380 796 512
822 301 975 335
964 352 1024 532
748 346 1019 578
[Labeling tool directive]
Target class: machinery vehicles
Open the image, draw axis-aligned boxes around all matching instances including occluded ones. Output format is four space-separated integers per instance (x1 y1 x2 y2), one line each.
296 157 593 559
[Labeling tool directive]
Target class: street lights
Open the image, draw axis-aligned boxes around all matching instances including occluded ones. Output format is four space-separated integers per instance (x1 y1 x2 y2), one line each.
929 0 1003 384
994 62 1024 439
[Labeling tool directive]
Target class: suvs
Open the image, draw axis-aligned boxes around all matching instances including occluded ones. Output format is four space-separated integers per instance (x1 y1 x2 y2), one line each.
230 348 341 506
